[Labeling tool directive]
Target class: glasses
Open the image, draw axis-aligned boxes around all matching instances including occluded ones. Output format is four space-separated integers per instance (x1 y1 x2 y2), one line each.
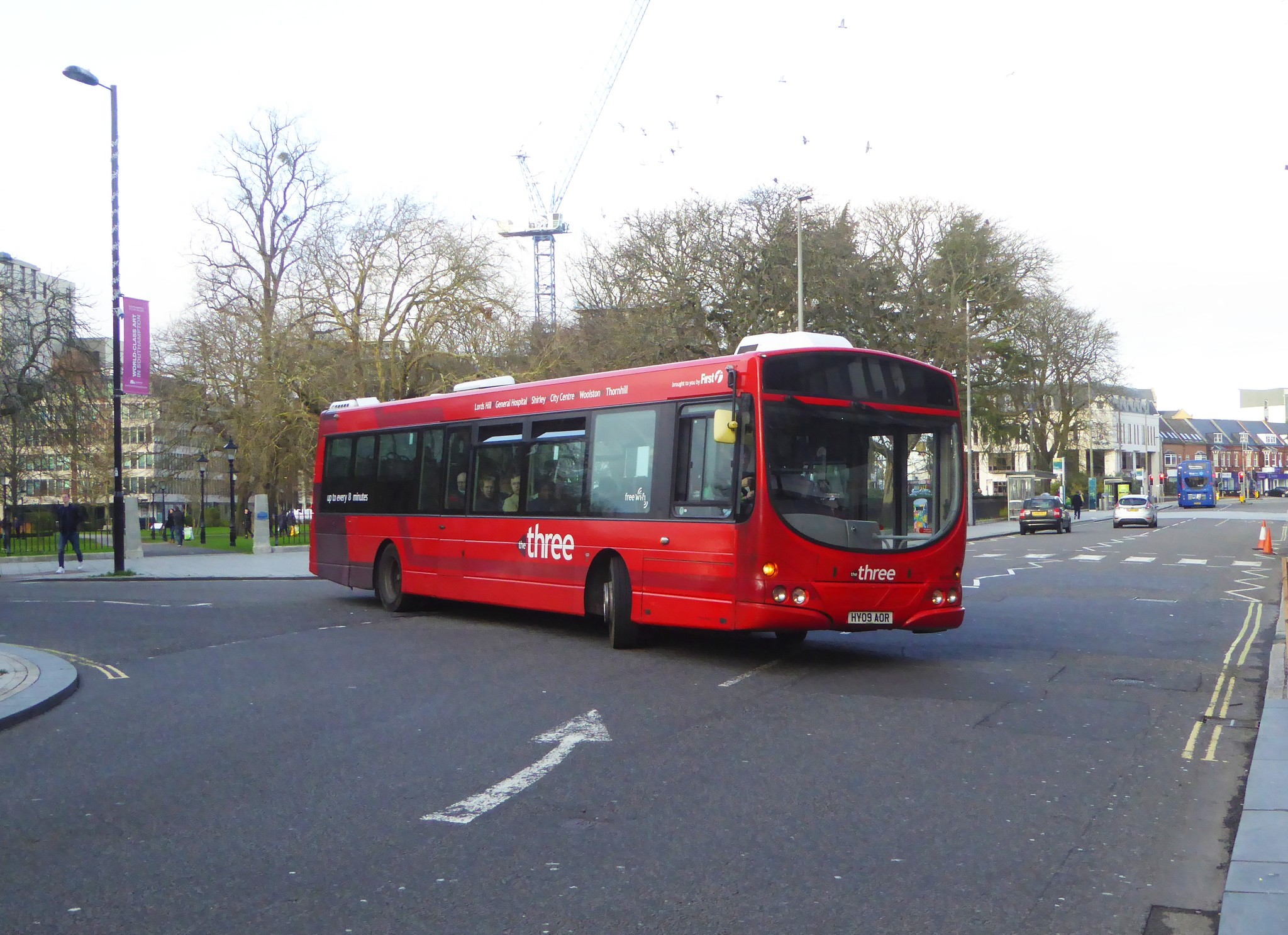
456 481 466 485
62 496 69 499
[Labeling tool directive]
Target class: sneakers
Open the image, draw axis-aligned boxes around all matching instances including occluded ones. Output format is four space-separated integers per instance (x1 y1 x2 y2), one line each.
55 566 65 574
77 560 84 570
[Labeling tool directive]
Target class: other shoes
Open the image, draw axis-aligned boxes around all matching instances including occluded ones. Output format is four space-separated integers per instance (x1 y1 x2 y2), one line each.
250 534 254 539
244 534 248 539
176 543 181 547
1074 515 1076 519
1077 518 1079 520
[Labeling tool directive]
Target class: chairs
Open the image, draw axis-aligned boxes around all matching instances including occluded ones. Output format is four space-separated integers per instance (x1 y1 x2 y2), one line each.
357 451 409 479
773 474 816 493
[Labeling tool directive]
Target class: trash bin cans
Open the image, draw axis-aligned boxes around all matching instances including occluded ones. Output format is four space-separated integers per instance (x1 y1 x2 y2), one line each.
913 498 929 533
1101 495 1109 511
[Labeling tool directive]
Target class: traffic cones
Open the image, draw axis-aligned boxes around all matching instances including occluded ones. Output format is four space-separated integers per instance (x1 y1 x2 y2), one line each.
1252 521 1266 550
1259 527 1276 555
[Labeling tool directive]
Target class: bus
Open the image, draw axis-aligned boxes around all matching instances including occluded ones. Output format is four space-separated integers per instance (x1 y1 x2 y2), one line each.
1177 460 1218 508
308 330 969 649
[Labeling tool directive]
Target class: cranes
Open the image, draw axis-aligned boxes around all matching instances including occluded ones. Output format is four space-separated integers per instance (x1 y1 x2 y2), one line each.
498 0 651 337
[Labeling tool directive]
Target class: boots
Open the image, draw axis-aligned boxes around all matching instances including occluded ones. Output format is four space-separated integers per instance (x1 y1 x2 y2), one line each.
170 539 175 543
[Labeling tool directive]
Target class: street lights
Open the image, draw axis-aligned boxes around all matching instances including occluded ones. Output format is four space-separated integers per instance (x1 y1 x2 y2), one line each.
150 484 156 539
965 298 978 525
196 453 210 543
796 194 814 330
63 65 128 574
222 435 241 547
159 480 166 540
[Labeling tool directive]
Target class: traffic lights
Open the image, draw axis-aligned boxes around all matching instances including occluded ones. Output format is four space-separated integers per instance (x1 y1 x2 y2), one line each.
1159 474 1164 485
1239 472 1244 483
1148 475 1153 486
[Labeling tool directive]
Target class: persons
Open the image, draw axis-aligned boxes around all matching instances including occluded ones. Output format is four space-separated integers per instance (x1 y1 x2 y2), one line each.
1072 491 1084 519
0 515 20 550
164 506 189 547
446 459 620 518
57 493 84 574
243 508 297 538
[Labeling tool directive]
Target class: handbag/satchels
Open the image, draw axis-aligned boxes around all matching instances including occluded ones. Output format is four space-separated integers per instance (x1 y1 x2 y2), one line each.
295 525 299 535
184 527 194 540
290 526 296 536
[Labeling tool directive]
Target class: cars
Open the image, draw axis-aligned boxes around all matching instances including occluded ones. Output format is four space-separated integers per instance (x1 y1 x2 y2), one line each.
1019 496 1071 535
1264 487 1288 498
1113 494 1159 528
909 489 930 496
